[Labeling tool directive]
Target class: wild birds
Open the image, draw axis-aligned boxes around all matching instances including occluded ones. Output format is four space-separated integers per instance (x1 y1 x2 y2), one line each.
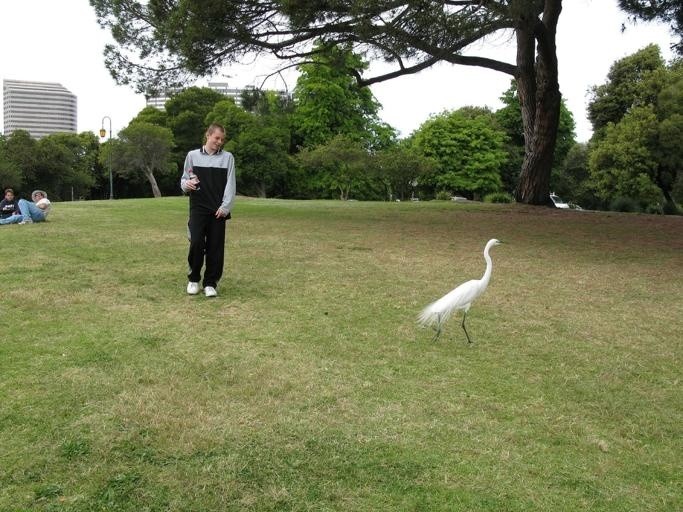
415 238 511 344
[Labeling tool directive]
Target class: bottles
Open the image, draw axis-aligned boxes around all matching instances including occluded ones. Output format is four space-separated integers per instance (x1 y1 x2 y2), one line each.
187 169 200 191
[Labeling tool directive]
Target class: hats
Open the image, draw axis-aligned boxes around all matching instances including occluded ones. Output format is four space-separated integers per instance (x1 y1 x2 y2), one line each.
32 190 47 201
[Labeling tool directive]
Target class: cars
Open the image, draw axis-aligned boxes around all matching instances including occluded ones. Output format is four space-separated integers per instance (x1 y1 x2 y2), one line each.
450 195 466 201
547 192 569 209
393 199 400 202
409 198 420 202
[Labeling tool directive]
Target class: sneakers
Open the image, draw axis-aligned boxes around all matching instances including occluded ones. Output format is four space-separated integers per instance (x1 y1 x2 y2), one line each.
204 286 217 297
187 282 199 294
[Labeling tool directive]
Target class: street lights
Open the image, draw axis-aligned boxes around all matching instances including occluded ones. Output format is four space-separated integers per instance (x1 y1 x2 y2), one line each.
99 116 114 200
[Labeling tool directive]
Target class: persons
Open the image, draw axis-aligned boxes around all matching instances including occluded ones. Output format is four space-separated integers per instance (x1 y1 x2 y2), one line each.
180 123 237 297
0 190 51 225
0 188 21 219
